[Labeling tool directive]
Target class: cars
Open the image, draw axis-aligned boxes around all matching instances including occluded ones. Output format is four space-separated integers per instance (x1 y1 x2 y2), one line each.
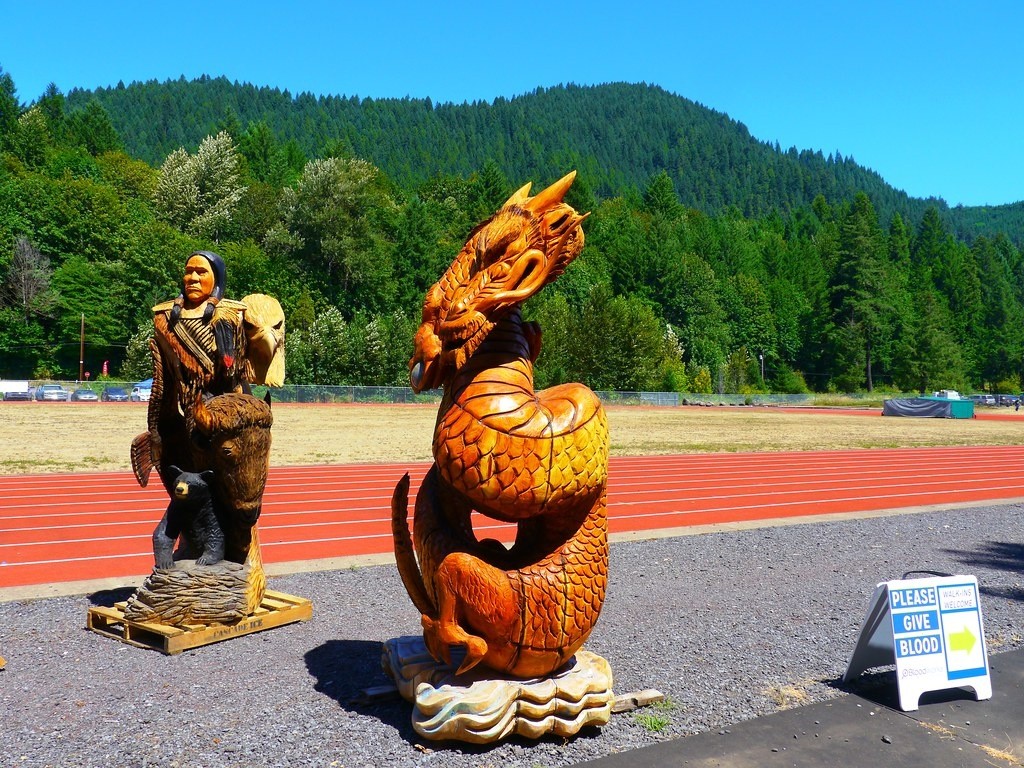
101 386 129 402
35 384 68 401
3 384 34 401
71 389 99 401
960 394 1024 407
130 387 151 402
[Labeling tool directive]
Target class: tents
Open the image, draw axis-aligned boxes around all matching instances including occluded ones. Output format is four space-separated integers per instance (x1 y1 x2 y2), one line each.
882 396 974 419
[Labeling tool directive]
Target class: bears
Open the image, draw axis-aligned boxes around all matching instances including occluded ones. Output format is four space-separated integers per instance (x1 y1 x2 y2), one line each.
151 465 226 568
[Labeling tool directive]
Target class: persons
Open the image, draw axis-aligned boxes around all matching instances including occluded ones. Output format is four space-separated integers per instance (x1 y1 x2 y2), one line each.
152 251 249 474
1015 397 1020 411
